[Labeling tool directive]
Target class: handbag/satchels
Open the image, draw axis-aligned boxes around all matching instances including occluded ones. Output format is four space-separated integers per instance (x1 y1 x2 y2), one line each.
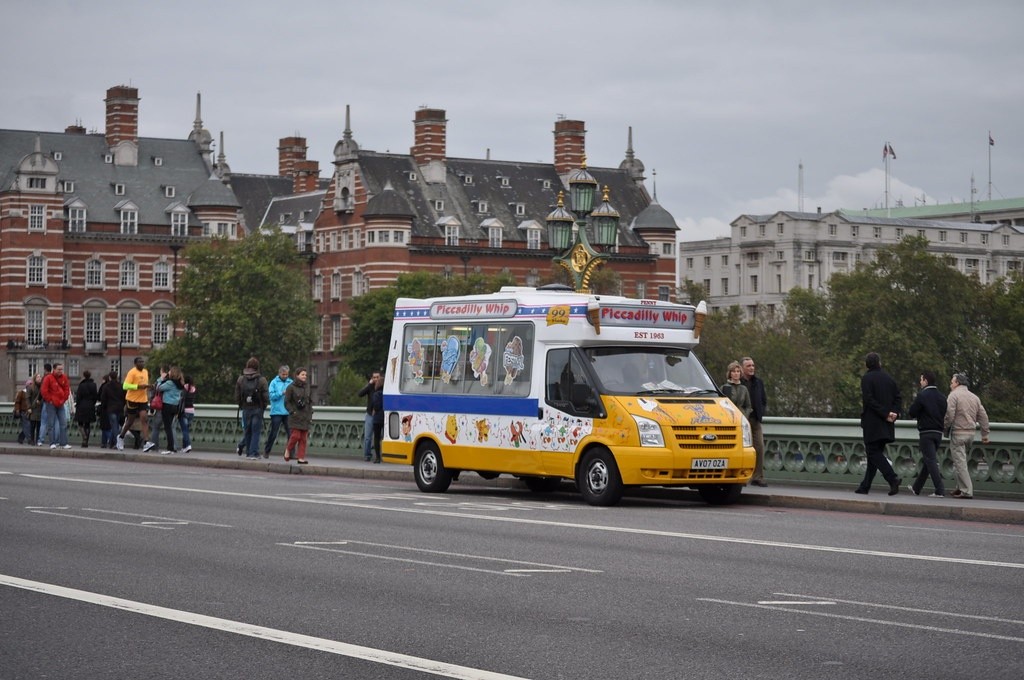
68 389 76 415
25 408 32 420
13 408 21 419
151 385 163 410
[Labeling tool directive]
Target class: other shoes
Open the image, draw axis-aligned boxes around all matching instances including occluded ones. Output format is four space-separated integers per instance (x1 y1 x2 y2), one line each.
948 490 972 499
15 435 116 449
161 450 174 455
297 461 308 464
855 487 868 494
284 447 289 461
751 476 768 487
181 445 191 453
134 436 142 449
365 457 370 461
908 484 917 496
888 477 901 495
236 444 269 459
928 493 944 497
374 458 380 463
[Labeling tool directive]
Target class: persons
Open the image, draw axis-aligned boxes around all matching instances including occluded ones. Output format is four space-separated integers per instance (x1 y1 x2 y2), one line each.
262 365 300 459
855 353 903 496
907 369 947 497
283 367 314 464
236 358 269 460
237 408 247 455
943 374 991 499
372 376 385 464
358 371 386 462
739 357 768 486
718 362 752 420
14 357 195 454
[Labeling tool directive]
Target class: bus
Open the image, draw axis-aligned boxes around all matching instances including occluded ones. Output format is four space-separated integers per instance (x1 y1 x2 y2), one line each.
379 282 757 505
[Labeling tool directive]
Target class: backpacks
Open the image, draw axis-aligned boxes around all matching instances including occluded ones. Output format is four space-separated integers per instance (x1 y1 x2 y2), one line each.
240 376 259 405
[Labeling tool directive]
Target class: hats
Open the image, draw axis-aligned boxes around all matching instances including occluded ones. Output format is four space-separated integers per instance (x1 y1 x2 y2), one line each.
25 380 32 388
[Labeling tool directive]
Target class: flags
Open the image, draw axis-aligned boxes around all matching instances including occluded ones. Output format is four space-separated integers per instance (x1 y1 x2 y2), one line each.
989 137 995 146
883 145 897 160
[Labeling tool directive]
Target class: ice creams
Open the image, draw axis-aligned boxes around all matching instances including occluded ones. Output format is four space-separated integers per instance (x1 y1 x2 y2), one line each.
694 300 708 339
588 295 601 334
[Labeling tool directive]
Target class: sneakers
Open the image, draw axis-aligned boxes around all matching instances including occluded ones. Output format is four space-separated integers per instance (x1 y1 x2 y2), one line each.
143 442 155 452
116 434 124 450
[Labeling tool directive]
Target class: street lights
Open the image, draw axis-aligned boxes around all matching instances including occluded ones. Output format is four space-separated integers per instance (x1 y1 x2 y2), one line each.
460 255 470 282
168 243 183 338
308 251 318 400
